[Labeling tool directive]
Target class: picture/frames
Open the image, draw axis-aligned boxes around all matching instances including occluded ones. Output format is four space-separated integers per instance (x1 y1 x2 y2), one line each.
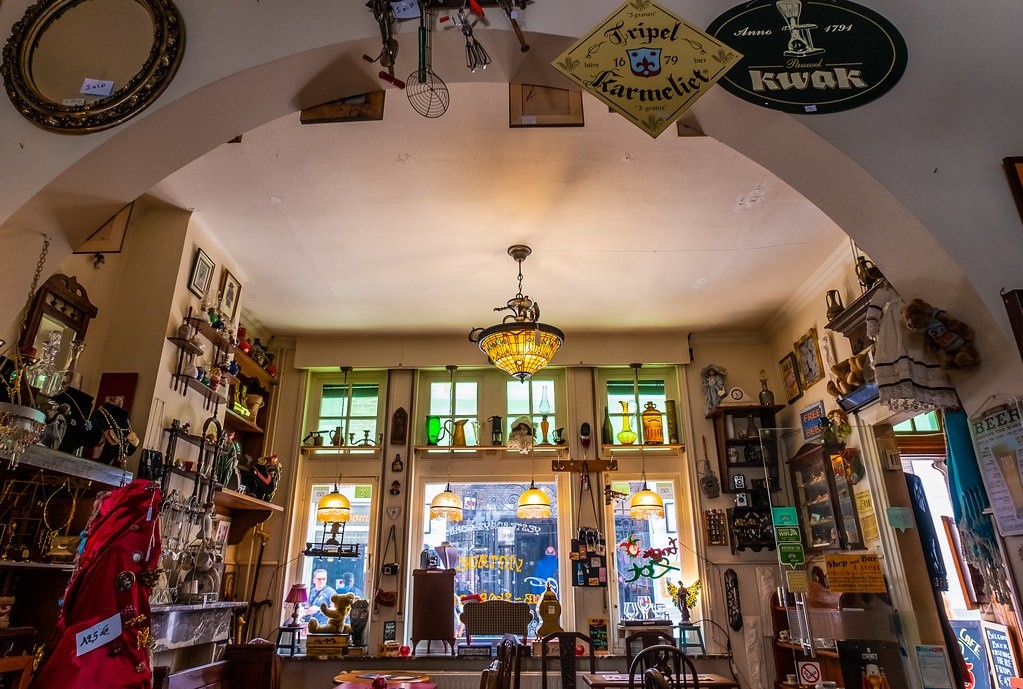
507 81 585 129
979 620 1020 689
299 88 387 125
793 328 824 391
214 520 232 545
778 351 804 405
217 267 242 324
797 399 826 441
186 247 216 302
72 200 134 254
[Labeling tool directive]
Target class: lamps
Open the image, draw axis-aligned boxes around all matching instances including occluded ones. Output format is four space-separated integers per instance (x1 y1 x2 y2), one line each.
429 365 463 523
518 374 551 520
629 363 666 520
468 245 565 384
284 583 308 627
316 366 354 523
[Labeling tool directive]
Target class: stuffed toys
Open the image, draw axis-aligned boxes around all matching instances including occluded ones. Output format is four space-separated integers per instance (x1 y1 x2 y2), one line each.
826 353 875 397
900 298 982 370
829 409 851 440
308 592 360 633
839 449 865 485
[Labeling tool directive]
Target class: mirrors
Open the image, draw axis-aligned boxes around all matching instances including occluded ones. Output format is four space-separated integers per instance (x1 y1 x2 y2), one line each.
0 0 185 134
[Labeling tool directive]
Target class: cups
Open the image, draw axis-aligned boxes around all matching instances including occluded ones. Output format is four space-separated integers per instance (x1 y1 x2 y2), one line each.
728 447 737 463
866 664 882 689
472 420 485 447
786 674 796 682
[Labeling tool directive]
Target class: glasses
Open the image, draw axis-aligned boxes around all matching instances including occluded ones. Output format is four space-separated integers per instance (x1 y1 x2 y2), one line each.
314 577 326 581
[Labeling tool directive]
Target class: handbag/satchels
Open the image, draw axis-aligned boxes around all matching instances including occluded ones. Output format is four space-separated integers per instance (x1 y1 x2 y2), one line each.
375 589 398 607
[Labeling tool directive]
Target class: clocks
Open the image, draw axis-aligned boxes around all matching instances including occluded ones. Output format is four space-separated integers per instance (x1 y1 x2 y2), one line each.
720 386 758 406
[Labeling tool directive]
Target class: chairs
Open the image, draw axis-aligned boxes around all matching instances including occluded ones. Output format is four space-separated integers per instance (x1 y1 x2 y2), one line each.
625 631 677 675
542 632 595 689
628 644 700 689
497 633 521 689
480 657 504 689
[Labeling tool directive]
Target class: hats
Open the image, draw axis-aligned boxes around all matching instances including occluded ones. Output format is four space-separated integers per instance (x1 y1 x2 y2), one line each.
342 572 355 581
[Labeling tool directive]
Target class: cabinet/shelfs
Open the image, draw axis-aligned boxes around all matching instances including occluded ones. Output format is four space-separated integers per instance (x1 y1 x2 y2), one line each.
785 443 868 552
769 588 909 689
705 403 786 495
0 435 134 570
166 306 284 513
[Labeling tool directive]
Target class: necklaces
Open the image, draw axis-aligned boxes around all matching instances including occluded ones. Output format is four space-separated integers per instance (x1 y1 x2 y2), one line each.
63 391 93 430
98 406 133 462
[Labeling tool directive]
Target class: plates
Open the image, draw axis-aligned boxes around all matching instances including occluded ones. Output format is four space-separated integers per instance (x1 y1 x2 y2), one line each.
783 681 798 685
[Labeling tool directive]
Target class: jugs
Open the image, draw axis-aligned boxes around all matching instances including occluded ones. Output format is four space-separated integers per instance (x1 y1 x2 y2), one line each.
444 419 469 445
617 399 638 445
552 427 566 446
424 415 445 445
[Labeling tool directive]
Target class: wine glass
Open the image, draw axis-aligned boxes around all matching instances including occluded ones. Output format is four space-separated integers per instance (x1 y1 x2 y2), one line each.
624 595 667 621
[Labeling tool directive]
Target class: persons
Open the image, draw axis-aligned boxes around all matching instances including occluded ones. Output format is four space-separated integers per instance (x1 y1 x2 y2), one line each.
52 403 70 447
454 595 465 637
302 569 337 636
211 369 222 390
532 547 559 622
676 581 690 622
336 572 362 624
702 364 729 413
30 330 61 388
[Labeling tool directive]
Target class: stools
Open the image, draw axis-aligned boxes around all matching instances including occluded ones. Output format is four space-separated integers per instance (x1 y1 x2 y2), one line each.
678 625 706 655
274 626 303 655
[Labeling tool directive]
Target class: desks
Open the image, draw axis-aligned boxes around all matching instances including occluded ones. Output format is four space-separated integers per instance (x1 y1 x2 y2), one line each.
334 681 438 689
581 673 740 689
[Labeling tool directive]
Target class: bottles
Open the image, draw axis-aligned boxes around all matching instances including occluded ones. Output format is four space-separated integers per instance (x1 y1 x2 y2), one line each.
759 379 774 405
183 353 211 386
177 323 190 339
237 327 277 377
578 563 584 585
746 415 759 439
603 406 613 444
642 401 664 444
